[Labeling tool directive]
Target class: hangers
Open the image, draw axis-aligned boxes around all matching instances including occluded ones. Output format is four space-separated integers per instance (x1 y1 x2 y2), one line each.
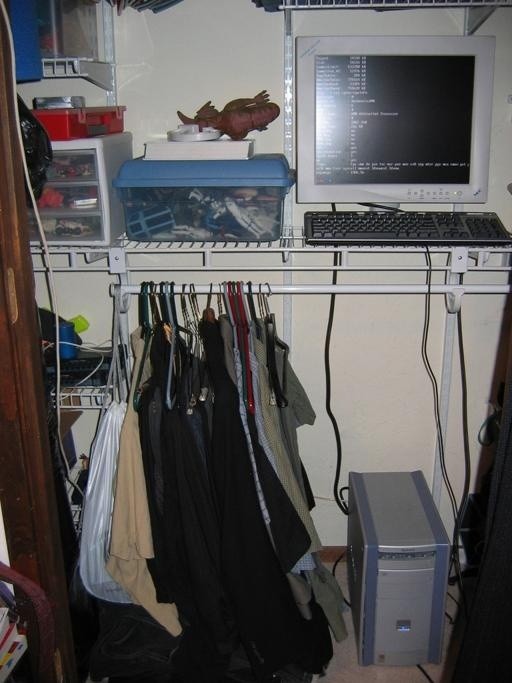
133 280 274 335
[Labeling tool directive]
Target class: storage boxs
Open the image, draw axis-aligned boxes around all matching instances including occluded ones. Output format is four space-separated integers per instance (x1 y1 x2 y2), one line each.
111 150 296 242
29 105 127 139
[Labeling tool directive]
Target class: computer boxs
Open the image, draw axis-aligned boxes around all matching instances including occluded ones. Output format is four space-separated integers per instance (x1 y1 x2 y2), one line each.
348 472 451 666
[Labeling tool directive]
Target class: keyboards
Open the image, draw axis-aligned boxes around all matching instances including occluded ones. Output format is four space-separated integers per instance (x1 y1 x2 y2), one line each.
302 211 512 247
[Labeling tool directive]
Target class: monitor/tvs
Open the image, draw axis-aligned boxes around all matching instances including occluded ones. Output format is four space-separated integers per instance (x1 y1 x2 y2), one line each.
293 34 497 205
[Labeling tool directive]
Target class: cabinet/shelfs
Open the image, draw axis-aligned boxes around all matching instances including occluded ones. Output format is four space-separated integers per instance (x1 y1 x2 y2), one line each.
20 133 136 247
